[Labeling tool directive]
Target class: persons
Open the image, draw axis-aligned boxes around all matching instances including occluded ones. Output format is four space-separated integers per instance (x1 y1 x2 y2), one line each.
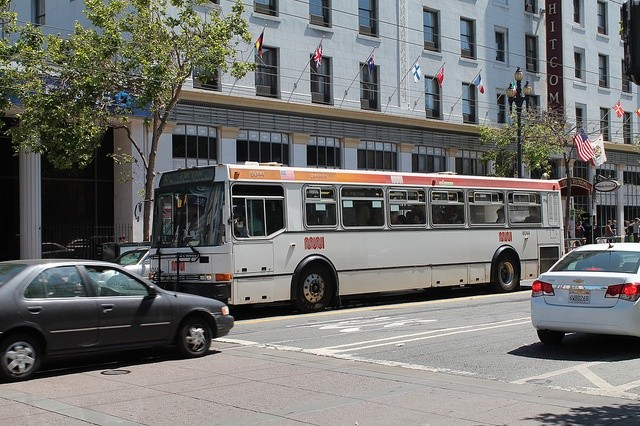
524 206 540 223
391 210 425 225
236 213 263 237
434 209 463 225
495 209 505 224
575 217 640 243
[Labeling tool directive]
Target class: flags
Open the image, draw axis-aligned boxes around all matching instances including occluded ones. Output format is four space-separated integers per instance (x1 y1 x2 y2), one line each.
367 56 376 74
590 135 607 166
313 45 323 70
574 128 594 164
506 80 524 98
412 62 422 84
254 34 264 59
636 109 640 117
612 101 624 118
474 75 484 95
436 66 445 89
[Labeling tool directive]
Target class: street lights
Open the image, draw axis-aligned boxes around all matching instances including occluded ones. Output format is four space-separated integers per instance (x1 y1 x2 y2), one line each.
505 65 532 178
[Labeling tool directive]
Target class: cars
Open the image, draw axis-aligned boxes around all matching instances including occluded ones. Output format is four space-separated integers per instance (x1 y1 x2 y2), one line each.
115 248 152 279
42 241 70 259
0 258 236 381
65 238 91 255
530 240 640 356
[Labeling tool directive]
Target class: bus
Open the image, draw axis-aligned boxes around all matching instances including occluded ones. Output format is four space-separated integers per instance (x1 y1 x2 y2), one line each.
153 162 567 311
